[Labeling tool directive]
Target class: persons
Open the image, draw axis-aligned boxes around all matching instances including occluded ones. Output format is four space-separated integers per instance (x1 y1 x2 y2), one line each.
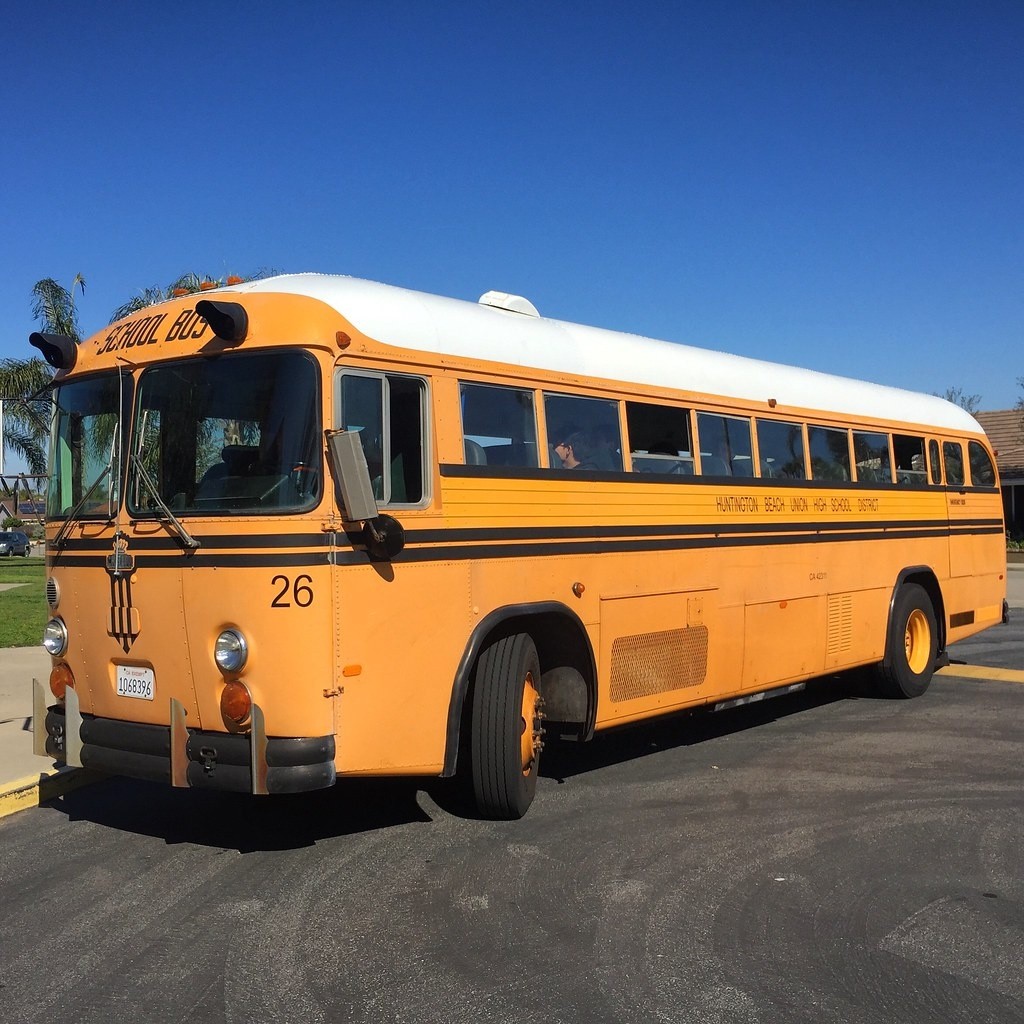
552 420 601 472
588 423 623 472
712 436 747 477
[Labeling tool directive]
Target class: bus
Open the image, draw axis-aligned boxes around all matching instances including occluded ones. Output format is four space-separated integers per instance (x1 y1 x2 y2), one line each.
35 272 1013 819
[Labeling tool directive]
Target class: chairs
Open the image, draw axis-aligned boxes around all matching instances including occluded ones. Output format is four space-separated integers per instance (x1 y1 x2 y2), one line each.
463 435 911 483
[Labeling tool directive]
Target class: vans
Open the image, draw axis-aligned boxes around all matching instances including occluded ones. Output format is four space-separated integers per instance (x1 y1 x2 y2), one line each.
0 531 32 559
